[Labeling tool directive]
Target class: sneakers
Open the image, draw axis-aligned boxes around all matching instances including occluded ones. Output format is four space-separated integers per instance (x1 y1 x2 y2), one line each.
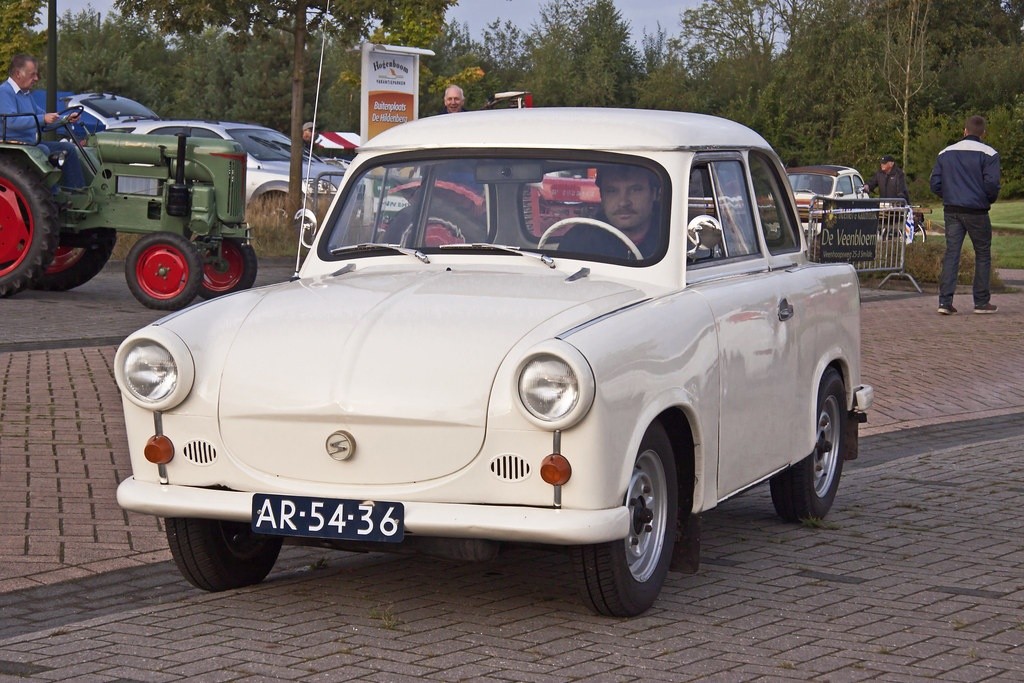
937 305 959 315
973 301 998 314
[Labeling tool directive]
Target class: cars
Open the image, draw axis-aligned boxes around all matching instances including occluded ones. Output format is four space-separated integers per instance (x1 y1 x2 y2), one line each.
118 106 873 617
766 164 871 223
58 92 365 220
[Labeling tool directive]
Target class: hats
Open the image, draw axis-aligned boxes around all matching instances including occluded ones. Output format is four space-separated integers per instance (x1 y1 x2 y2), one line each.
301 121 317 131
880 155 896 163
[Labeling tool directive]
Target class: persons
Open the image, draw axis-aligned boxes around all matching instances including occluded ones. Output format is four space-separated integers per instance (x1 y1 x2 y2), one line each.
0 55 86 190
861 154 910 210
556 166 667 261
929 117 1001 316
440 84 468 112
302 121 317 150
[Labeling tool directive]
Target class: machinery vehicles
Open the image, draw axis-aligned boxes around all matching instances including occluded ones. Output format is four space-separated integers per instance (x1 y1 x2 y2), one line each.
0 102 260 310
373 165 600 255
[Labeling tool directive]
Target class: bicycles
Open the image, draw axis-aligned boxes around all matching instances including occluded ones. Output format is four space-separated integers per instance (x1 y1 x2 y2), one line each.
857 186 926 250
